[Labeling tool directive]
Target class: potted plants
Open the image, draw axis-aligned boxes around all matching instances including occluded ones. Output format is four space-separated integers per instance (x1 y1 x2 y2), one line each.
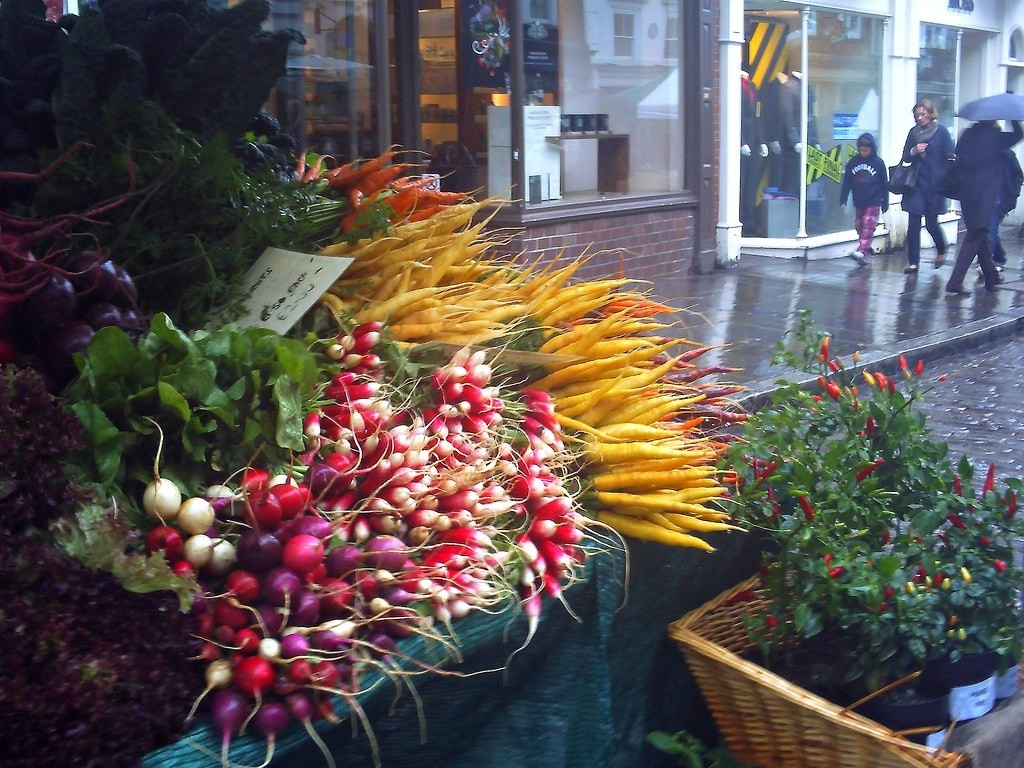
714 306 1024 746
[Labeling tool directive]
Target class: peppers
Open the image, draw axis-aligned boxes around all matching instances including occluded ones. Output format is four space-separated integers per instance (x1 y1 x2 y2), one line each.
713 334 1018 642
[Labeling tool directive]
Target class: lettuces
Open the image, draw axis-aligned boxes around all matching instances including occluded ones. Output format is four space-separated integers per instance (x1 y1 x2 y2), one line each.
0 363 210 768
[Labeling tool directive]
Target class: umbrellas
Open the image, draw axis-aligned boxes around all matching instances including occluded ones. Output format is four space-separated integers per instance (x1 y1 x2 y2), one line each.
955 93 1024 121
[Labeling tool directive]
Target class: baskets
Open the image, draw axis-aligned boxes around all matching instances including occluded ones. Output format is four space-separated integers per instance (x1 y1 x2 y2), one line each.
666 528 1024 768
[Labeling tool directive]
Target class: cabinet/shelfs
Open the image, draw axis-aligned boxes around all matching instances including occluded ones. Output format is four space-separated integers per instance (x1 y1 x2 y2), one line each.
487 106 561 202
546 133 630 197
525 21 559 91
387 9 458 163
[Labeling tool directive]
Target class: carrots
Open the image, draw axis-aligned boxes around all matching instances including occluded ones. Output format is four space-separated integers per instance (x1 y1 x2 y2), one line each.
293 150 755 553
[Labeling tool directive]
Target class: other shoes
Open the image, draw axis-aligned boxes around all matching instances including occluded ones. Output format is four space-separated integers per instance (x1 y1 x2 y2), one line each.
935 254 946 269
904 263 919 274
986 276 1005 289
851 251 871 265
996 265 1007 274
946 287 972 295
975 266 984 276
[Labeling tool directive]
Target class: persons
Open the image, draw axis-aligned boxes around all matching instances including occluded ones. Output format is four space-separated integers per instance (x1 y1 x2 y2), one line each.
946 120 1024 295
900 99 954 274
839 133 889 264
740 69 817 235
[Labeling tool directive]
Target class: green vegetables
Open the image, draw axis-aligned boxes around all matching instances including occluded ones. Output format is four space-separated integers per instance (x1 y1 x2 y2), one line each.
62 312 317 522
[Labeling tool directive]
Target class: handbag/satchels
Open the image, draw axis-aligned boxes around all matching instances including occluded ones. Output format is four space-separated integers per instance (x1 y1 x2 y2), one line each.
889 157 920 194
931 153 960 200
